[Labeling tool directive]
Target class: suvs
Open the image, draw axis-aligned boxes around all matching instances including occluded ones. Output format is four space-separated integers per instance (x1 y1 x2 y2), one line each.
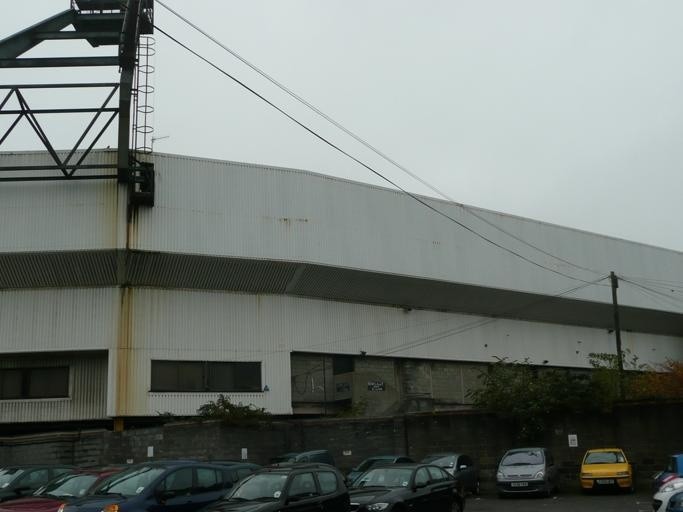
579 447 634 494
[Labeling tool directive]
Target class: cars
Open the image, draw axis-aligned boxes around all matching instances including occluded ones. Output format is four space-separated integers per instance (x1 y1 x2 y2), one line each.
58 459 257 509
268 450 335 466
420 453 481 500
206 462 346 508
649 452 683 512
0 466 79 501
0 466 127 509
346 463 467 510
347 456 415 487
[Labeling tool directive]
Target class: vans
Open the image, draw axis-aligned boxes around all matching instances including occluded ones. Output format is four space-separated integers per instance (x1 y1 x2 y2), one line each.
495 447 559 498
